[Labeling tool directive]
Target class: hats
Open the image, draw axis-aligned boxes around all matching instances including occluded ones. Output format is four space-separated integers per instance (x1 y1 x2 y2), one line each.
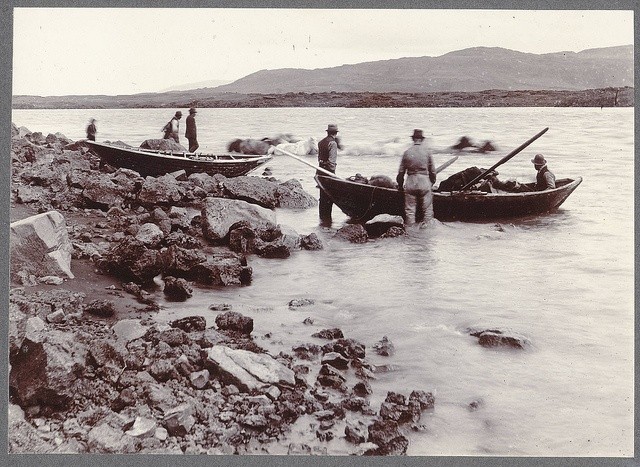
410 129 425 139
189 108 197 113
175 112 182 116
531 154 547 166
325 124 339 132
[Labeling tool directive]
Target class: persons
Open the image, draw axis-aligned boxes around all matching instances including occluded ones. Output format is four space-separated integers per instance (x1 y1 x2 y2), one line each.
530 153 556 193
315 122 340 221
395 128 437 226
164 110 183 143
85 118 97 142
184 107 199 153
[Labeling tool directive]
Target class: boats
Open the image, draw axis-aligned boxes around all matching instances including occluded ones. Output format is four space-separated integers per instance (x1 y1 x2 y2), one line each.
82 139 275 179
312 172 584 223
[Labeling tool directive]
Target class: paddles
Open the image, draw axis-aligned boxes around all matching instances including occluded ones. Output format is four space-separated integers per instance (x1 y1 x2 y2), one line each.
434 156 459 174
271 143 344 181
458 126 550 193
